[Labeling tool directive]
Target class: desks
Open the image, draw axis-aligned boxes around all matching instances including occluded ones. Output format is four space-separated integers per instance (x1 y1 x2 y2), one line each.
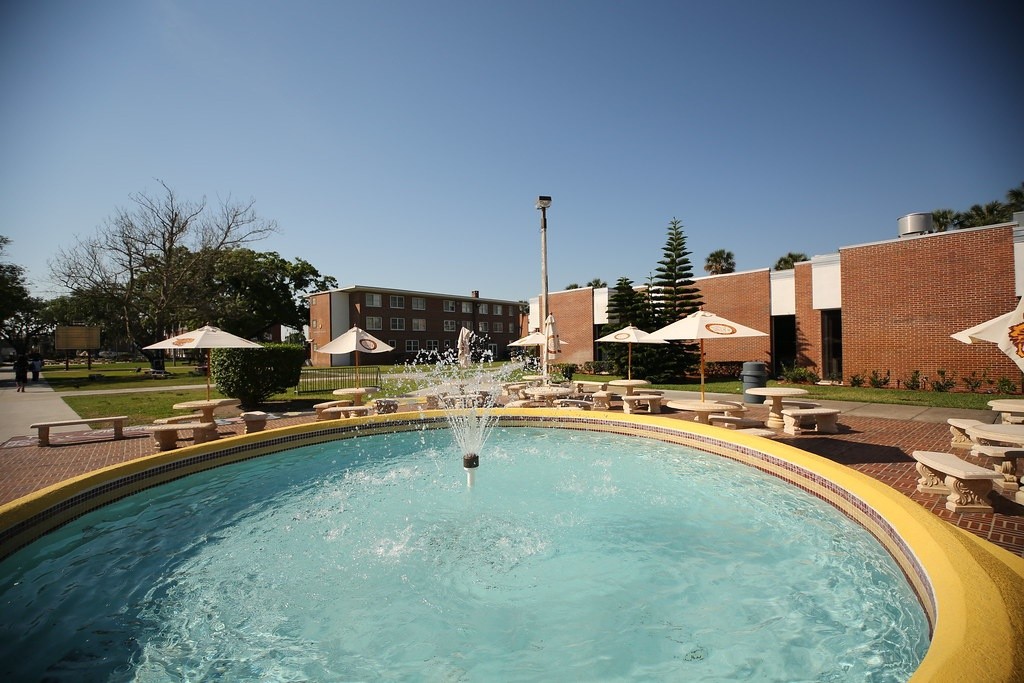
666 399 742 424
522 375 552 386
967 398 1024 505
745 388 809 428
525 387 574 407
333 387 381 418
609 380 651 396
173 398 241 441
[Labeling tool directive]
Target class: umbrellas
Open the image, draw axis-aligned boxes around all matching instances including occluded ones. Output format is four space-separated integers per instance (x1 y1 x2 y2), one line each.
594 323 670 380
950 295 1024 374
545 311 561 388
507 331 569 375
141 324 265 401
456 326 470 368
316 323 395 388
650 305 770 403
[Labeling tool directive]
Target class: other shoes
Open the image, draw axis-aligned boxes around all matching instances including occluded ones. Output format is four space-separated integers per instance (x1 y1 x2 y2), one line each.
17 387 20 392
22 390 25 392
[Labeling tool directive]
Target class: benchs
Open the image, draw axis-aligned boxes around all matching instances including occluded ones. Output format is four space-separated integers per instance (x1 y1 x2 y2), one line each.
913 451 1005 513
148 411 268 452
30 415 128 447
947 419 984 448
313 382 841 435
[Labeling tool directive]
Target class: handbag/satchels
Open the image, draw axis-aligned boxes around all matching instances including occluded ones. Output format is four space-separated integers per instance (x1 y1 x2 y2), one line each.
29 361 35 370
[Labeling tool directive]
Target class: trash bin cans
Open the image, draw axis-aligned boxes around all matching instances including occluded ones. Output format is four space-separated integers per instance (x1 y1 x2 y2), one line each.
740 361 767 403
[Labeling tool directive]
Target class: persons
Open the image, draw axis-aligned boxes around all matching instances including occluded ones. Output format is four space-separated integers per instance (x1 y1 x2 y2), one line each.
13 355 41 392
